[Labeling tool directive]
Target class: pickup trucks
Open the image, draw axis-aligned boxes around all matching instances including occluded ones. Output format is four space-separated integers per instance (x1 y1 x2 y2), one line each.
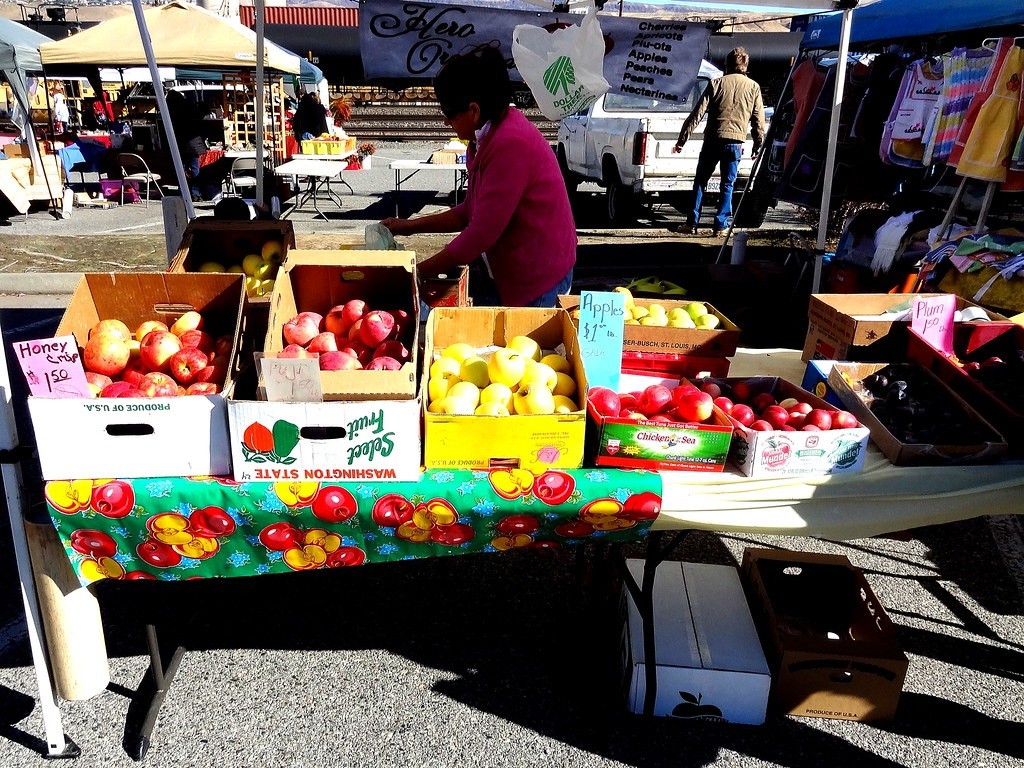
554 58 781 227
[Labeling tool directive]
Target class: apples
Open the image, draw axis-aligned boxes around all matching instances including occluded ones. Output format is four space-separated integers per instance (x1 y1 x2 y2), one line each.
83 311 235 397
612 287 721 330
201 240 282 297
589 385 714 422
428 336 578 417
281 301 410 370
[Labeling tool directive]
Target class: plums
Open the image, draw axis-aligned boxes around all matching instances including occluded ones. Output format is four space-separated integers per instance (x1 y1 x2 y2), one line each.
700 382 858 430
867 349 1024 446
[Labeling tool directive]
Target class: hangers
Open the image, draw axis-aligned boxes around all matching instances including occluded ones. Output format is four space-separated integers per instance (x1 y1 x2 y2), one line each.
899 45 937 71
814 46 859 71
982 37 1024 53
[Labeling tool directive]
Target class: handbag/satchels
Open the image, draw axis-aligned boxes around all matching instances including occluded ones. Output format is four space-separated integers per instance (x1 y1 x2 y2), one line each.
512 7 612 121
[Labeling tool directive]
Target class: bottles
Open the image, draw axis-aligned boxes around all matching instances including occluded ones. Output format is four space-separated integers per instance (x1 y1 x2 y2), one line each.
730 232 749 265
123 122 130 136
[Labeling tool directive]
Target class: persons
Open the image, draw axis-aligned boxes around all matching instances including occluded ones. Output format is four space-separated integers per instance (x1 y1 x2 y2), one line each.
672 46 764 237
377 45 579 308
52 89 69 129
292 83 319 143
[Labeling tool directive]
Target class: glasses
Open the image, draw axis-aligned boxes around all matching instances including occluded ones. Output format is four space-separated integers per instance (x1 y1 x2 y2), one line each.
439 108 469 120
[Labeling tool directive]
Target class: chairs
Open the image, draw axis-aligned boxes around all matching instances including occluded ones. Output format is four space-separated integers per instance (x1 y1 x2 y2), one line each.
118 152 165 208
224 157 258 200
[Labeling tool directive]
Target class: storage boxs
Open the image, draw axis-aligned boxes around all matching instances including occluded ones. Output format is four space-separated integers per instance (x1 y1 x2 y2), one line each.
28 218 1024 486
427 148 469 165
621 558 771 725
738 546 909 721
345 137 357 151
300 137 346 155
99 179 139 203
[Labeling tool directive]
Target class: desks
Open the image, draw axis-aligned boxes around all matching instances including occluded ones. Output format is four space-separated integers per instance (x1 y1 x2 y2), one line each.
390 159 467 219
274 151 353 222
221 151 271 192
41 348 1024 759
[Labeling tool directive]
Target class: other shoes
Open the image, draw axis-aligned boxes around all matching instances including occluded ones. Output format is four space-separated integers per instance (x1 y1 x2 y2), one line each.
677 224 696 235
713 228 735 237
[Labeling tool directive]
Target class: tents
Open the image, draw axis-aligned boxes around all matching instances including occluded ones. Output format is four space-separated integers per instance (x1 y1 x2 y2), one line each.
37 0 301 187
176 39 323 133
716 0 1024 294
0 17 57 218
